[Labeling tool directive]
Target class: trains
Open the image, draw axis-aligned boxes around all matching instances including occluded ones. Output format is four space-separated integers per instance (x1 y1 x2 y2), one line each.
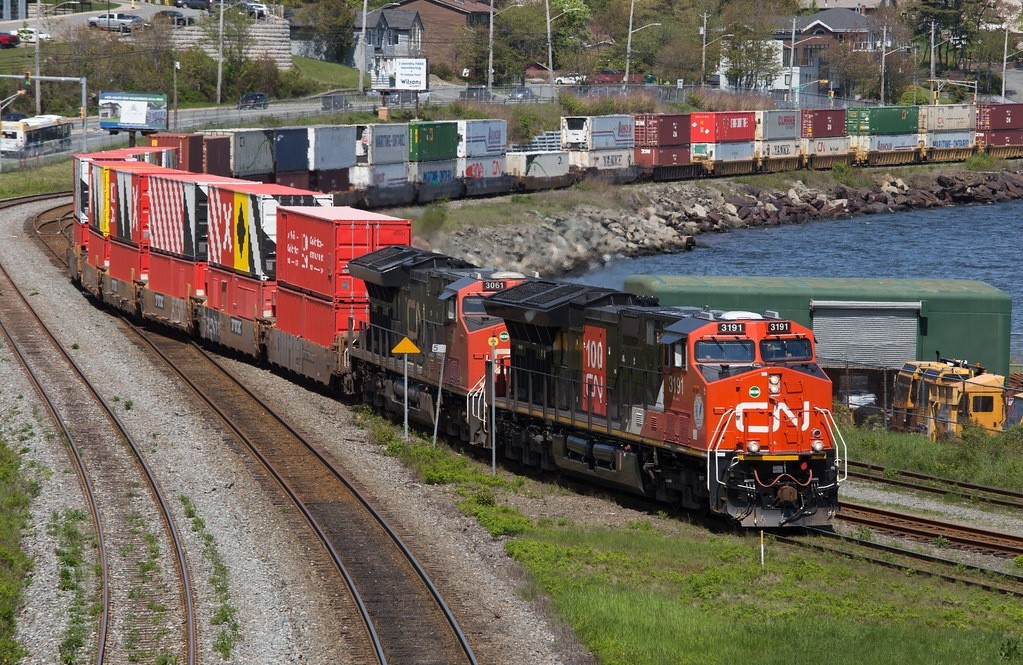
64 101 1023 536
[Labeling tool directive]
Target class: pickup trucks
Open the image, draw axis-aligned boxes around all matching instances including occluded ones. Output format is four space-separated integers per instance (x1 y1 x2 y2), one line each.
87 11 152 34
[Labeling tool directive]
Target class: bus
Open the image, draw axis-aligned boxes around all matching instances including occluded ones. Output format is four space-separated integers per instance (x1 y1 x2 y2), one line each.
0 113 74 159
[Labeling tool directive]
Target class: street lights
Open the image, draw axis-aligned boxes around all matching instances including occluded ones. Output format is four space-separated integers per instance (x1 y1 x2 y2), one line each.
625 22 662 82
215 1 249 102
358 3 401 89
929 38 955 105
700 34 734 84
547 7 581 83
881 46 913 105
488 4 523 90
788 36 823 101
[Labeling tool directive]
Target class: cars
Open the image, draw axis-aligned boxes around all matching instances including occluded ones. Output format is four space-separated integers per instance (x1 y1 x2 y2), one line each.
0 32 20 49
9 28 52 44
508 87 535 101
459 87 491 102
237 93 269 109
154 10 195 27
172 0 233 18
555 72 586 85
239 2 267 20
